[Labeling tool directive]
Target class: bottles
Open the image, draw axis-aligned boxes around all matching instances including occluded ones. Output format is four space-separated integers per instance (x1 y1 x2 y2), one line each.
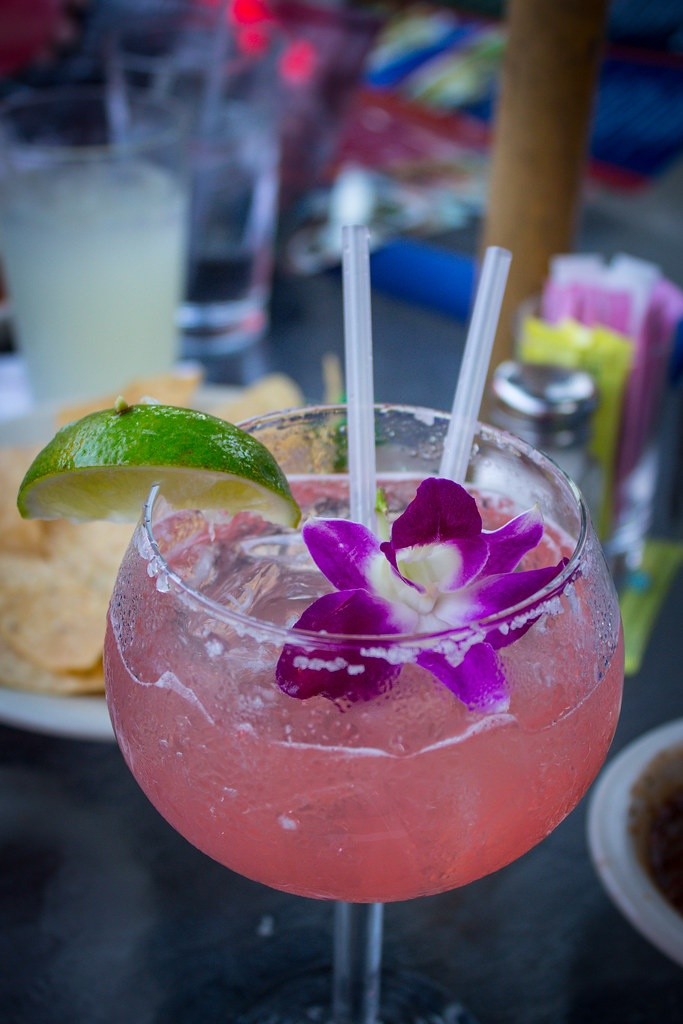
481 361 596 507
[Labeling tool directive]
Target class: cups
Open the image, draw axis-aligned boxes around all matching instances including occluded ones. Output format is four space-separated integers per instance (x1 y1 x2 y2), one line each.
0 93 184 417
106 28 277 356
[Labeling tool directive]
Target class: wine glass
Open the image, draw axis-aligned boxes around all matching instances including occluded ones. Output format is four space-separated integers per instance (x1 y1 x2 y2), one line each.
108 404 628 1024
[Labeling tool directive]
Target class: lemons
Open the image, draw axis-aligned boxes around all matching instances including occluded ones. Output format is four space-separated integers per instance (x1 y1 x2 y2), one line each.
17 403 303 529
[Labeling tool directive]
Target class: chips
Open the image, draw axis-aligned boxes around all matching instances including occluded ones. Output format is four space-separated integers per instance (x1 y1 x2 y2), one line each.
0 373 304 698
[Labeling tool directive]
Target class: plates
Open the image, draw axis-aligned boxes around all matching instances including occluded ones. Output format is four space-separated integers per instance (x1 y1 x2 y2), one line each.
0 384 245 740
588 722 683 960
